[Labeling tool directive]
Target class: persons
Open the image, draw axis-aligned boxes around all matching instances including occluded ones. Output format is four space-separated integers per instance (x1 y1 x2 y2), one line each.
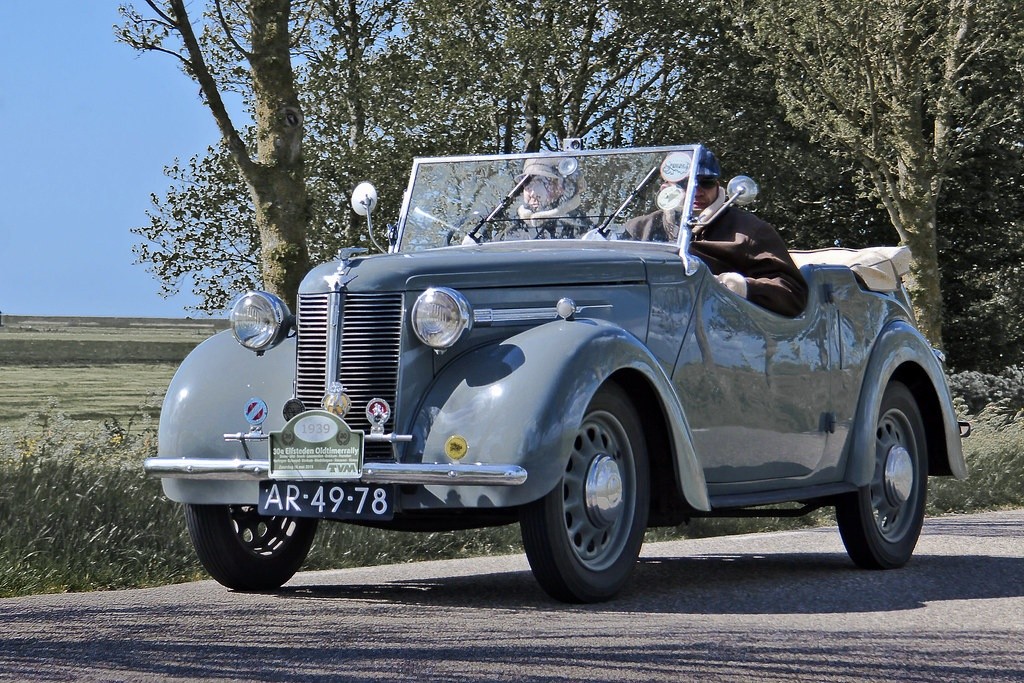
484 154 606 243
615 146 811 319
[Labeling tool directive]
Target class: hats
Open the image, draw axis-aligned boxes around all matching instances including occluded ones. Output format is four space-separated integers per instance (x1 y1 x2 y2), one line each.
514 150 587 194
665 144 720 183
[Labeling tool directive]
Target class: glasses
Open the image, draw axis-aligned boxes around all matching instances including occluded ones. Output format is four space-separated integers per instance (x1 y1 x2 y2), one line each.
677 175 718 190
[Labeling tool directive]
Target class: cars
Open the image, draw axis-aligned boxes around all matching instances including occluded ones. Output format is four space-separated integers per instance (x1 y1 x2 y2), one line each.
144 142 973 606
